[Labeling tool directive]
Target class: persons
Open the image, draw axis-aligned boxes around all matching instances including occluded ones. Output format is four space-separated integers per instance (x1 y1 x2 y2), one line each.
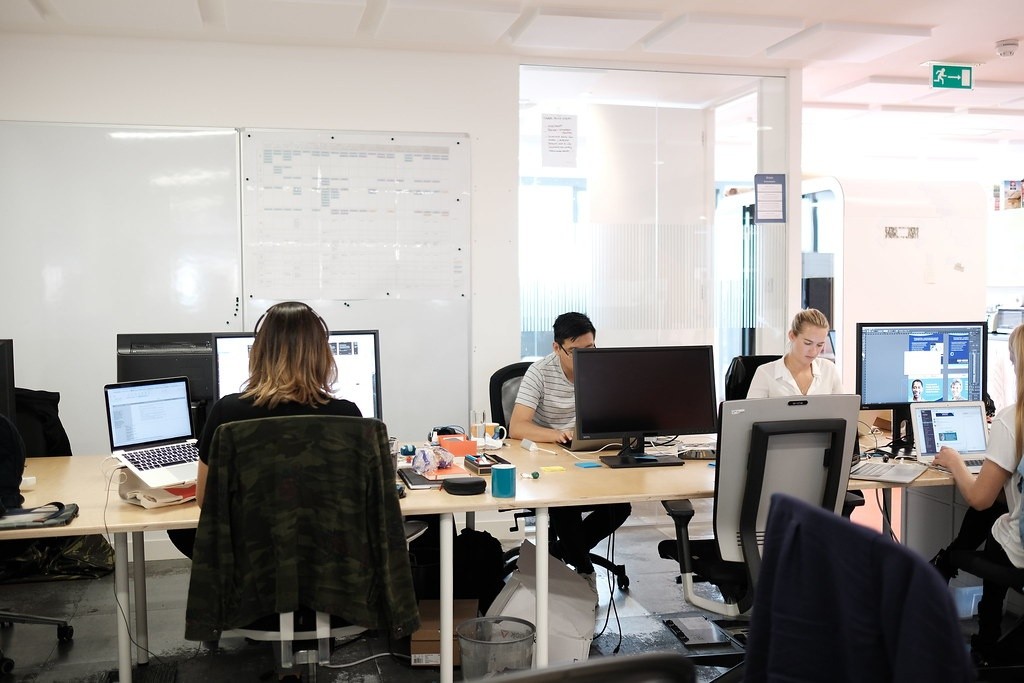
196 302 363 509
940 433 945 440
951 380 966 400
509 313 632 606
927 324 1024 646
910 380 925 401
746 308 844 399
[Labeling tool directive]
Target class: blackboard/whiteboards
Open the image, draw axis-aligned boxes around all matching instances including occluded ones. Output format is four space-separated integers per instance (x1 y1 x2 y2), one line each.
0 120 242 457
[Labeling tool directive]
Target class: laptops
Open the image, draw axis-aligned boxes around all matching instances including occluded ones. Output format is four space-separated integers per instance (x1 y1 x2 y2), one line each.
556 426 639 452
848 429 929 485
104 376 202 490
910 401 991 474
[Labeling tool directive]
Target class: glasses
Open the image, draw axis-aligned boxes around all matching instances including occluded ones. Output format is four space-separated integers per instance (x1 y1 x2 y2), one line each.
555 339 596 359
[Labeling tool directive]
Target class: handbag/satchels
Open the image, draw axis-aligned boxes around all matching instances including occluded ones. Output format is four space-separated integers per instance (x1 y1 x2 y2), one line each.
38 534 115 581
439 477 487 495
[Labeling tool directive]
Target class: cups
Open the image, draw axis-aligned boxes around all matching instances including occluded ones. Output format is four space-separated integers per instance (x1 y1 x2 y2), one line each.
491 464 516 498
469 409 487 449
19 476 37 489
388 436 398 482
484 423 507 442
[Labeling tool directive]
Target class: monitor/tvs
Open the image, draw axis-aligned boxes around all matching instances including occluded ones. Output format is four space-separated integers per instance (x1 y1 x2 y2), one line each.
854 322 989 459
215 331 383 420
573 345 718 469
116 330 214 443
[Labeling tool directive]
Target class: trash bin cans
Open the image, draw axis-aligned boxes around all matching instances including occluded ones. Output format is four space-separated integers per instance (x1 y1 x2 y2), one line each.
456 616 536 683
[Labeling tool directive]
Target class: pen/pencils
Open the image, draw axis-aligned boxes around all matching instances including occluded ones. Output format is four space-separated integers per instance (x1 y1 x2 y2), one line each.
503 443 511 447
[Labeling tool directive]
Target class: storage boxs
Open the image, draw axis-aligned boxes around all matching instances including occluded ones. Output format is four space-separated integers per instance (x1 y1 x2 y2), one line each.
947 573 1008 620
410 599 478 666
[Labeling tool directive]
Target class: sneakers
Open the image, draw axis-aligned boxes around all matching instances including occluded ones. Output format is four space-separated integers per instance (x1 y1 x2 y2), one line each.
580 571 598 608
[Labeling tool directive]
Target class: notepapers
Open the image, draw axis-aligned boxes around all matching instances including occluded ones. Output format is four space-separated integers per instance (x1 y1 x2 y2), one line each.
576 462 601 468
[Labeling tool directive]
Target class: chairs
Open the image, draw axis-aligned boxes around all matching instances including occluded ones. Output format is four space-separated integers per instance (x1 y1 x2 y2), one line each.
213 415 388 683
658 394 865 645
960 459 1024 668
489 360 630 589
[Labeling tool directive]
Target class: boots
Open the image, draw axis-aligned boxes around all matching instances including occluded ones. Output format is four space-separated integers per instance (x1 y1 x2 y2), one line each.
928 549 958 587
970 601 1002 644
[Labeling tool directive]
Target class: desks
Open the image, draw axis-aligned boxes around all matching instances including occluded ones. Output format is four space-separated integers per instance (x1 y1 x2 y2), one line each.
0 433 978 683
